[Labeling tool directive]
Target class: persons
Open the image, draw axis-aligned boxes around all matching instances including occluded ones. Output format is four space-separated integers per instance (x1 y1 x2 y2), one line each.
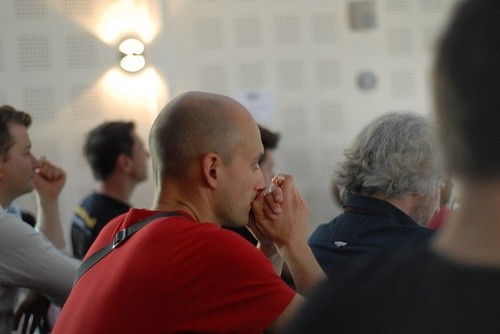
50 91 328 334
0 106 82 334
222 125 295 288
287 1 500 334
290 111 453 293
70 120 151 259
